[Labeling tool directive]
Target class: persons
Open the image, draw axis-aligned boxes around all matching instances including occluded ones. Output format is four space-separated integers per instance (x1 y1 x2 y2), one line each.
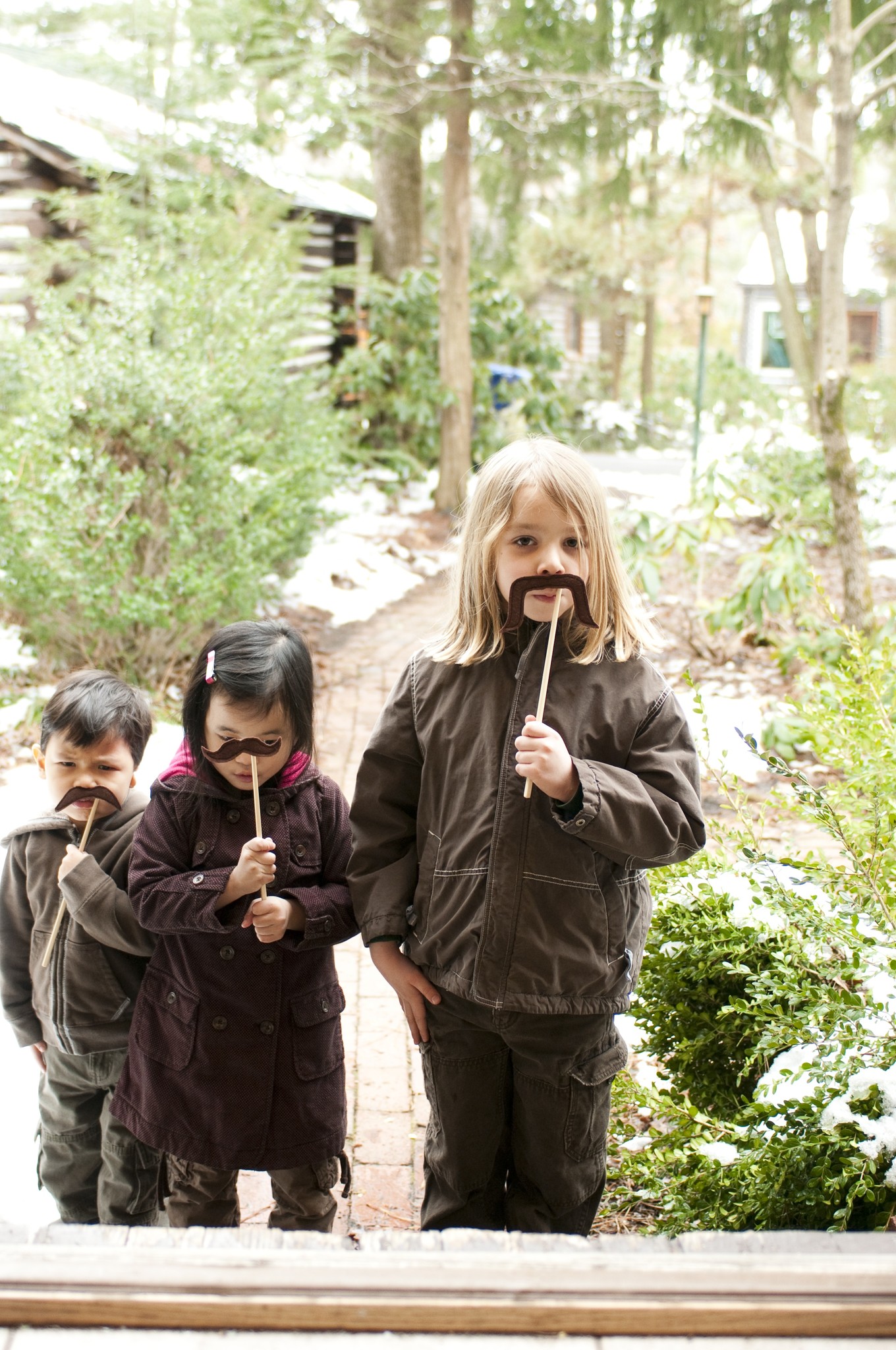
347 437 706 1235
107 620 361 1233
0 670 171 1227
